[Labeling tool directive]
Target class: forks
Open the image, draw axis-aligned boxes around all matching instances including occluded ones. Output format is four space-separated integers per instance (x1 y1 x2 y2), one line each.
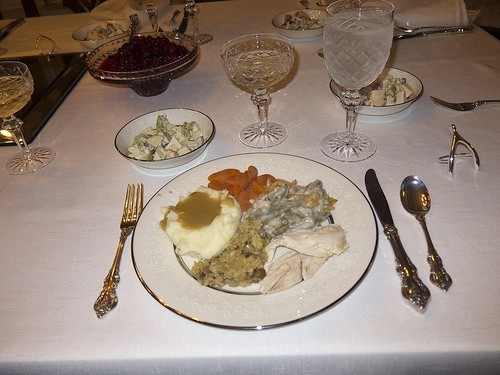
429 96 500 111
94 183 145 318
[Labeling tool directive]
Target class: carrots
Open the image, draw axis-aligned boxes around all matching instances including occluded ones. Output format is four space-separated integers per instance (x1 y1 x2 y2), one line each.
208 165 277 212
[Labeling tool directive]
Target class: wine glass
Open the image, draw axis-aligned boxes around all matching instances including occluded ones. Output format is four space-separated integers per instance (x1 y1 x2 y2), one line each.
130 1 213 45
220 32 295 148
0 61 57 175
319 0 395 162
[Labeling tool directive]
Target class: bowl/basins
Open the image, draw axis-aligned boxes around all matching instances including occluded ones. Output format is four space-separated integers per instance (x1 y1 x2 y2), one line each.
72 19 130 49
270 10 325 39
329 67 423 115
113 108 215 170
85 31 200 96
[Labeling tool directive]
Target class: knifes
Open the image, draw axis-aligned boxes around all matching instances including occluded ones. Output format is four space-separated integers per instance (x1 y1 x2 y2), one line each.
394 28 470 40
364 169 431 311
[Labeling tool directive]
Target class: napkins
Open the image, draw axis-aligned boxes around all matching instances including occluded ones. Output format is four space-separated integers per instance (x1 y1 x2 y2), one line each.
362 0 469 31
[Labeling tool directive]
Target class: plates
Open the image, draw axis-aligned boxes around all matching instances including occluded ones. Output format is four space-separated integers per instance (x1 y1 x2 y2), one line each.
130 152 380 331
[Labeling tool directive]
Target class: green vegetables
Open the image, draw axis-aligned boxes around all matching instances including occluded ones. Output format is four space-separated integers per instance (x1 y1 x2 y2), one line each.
136 114 194 161
288 15 308 30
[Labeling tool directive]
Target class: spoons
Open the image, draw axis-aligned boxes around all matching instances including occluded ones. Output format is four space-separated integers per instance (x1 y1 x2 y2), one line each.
393 25 471 33
400 176 453 292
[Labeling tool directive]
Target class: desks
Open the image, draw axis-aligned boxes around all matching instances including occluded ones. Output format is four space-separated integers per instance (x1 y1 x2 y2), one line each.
0 0 500 375
